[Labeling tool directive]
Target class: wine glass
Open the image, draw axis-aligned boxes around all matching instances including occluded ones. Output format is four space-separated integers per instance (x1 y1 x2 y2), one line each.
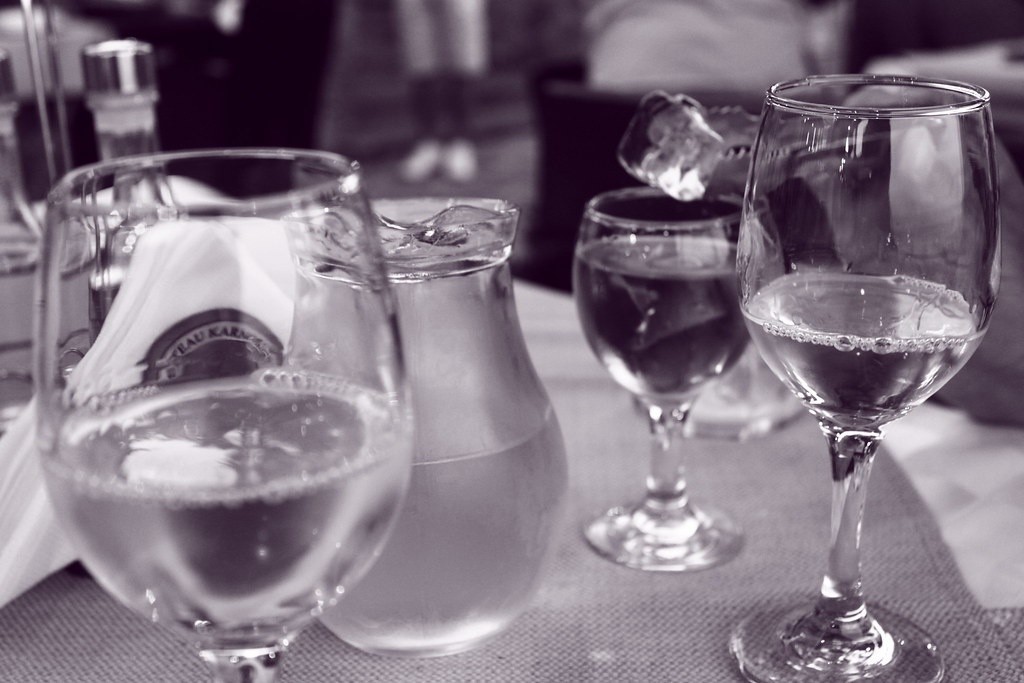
574 186 784 574
735 76 1001 683
40 148 413 683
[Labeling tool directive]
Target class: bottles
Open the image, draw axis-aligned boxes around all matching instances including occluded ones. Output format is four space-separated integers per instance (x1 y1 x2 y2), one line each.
0 48 41 409
80 39 193 350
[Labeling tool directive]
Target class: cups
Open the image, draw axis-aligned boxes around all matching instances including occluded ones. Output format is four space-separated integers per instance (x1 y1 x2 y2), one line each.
318 0 539 257
283 196 574 658
